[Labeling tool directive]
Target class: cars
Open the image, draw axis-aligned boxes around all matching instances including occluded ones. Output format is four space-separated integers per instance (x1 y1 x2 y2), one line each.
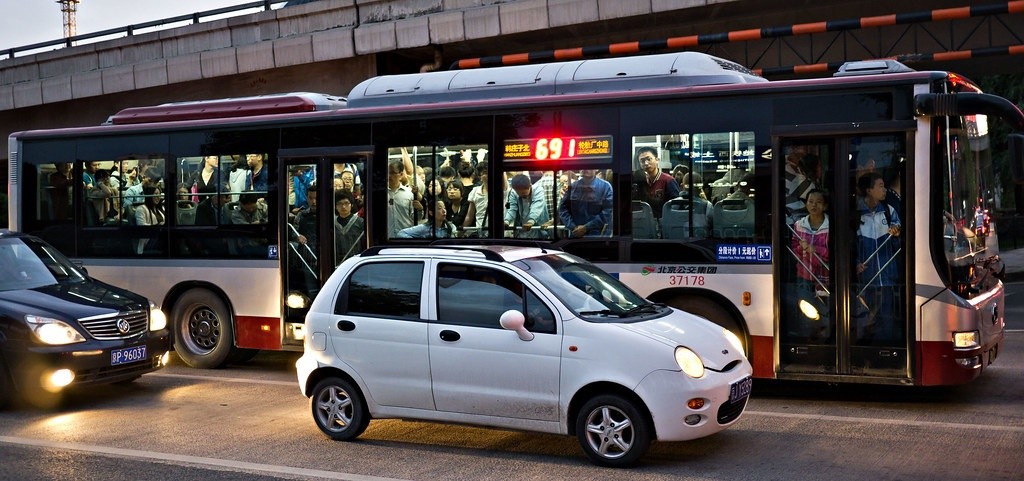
0 229 170 406
297 239 755 459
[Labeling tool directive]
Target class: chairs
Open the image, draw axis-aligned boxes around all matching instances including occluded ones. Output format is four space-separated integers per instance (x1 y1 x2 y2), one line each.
709 194 754 240
127 204 141 226
660 198 712 237
174 199 198 224
224 203 238 224
630 196 657 238
86 194 101 228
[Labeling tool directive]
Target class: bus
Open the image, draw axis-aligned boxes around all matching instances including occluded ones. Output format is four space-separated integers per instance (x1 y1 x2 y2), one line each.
5 52 1024 388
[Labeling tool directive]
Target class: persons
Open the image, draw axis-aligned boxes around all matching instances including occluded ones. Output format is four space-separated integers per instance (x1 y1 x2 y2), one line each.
783 141 834 345
386 145 751 240
44 153 272 227
555 272 589 311
286 163 369 292
973 205 989 241
854 145 903 345
